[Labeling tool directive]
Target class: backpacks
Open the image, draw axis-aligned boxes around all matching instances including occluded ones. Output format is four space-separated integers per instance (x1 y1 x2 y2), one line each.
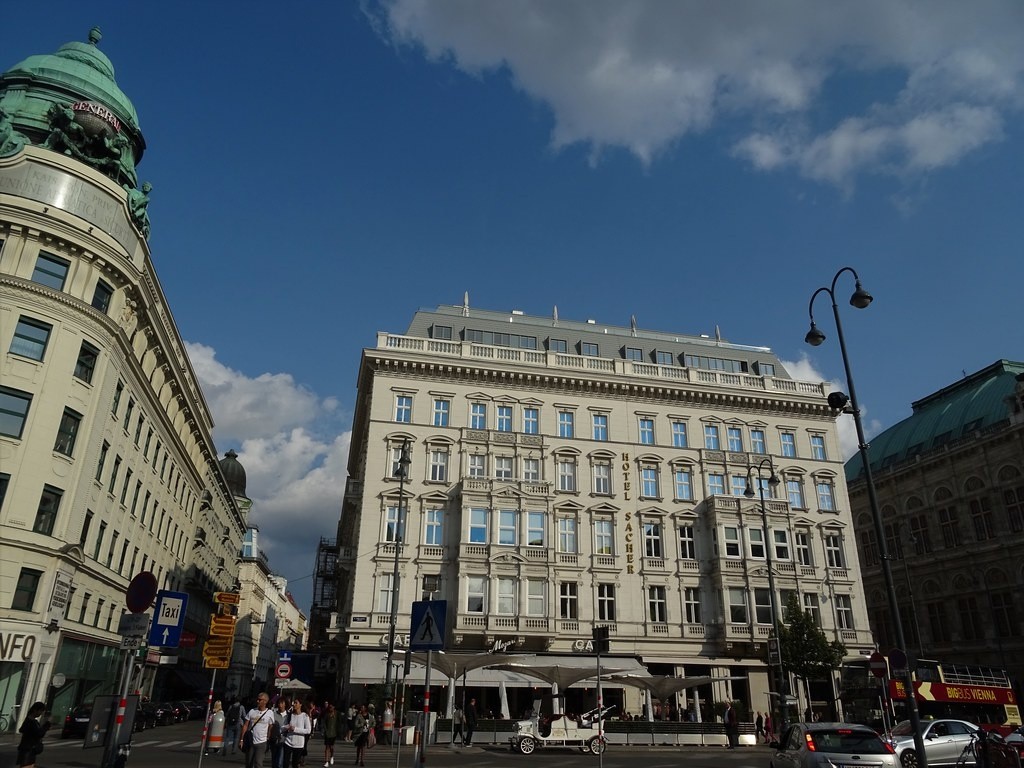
227 704 241 725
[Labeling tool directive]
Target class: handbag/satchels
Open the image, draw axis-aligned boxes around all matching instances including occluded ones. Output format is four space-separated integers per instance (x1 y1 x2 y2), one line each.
239 731 253 754
367 728 376 748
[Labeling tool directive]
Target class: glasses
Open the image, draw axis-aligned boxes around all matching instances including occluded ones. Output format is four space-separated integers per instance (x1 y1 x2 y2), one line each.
258 699 267 702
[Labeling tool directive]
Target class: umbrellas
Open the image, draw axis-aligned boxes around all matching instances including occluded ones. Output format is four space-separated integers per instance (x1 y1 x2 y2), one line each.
388 652 537 720
482 664 648 714
588 674 748 721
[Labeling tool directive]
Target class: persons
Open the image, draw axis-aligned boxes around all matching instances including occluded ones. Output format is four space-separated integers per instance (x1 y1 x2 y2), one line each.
206 693 394 768
756 711 779 744
16 702 51 768
453 698 477 746
725 701 737 749
619 703 695 722
104 135 127 184
128 180 152 229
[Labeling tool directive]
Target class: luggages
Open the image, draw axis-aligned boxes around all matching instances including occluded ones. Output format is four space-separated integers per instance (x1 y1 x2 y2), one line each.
204 753 208 756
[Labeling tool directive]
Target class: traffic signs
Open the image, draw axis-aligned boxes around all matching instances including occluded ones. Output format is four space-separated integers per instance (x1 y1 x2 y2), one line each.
204 591 240 669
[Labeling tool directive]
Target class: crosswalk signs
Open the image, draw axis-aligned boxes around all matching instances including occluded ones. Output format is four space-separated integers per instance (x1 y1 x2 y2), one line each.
405 597 448 652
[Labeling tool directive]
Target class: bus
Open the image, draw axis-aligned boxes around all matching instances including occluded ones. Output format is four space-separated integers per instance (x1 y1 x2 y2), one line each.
838 655 1021 728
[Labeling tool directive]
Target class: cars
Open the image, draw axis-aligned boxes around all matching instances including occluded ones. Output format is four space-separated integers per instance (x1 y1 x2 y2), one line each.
770 722 904 768
884 718 981 768
60 700 208 737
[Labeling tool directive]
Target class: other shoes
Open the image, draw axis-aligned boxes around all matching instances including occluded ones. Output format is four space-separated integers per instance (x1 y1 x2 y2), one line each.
355 760 359 765
214 749 220 753
323 762 329 768
726 746 734 749
465 745 472 748
360 761 364 766
344 737 353 742
329 756 334 764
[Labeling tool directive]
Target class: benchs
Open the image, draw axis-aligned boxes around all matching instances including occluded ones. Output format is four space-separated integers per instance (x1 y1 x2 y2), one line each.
543 714 578 729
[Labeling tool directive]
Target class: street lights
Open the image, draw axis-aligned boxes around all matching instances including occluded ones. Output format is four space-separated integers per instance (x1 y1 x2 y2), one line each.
382 439 413 707
744 459 791 744
805 265 928 768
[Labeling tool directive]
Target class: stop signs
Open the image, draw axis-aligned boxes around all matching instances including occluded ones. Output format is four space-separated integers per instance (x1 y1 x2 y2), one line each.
869 653 888 678
